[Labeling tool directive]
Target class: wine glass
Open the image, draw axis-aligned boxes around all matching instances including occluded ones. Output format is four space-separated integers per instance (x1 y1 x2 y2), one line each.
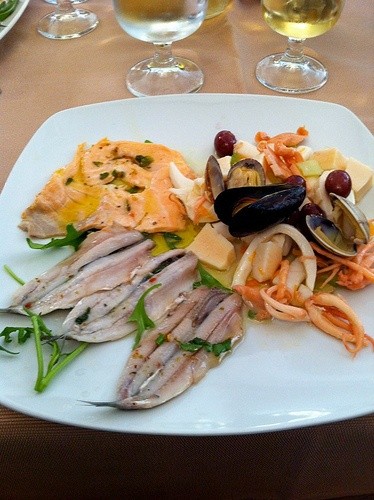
255 0 345 95
37 0 99 41
112 0 206 98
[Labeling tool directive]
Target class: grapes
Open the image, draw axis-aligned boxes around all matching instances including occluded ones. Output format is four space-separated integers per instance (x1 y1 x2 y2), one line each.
325 170 352 198
285 174 306 188
286 202 328 223
214 130 236 158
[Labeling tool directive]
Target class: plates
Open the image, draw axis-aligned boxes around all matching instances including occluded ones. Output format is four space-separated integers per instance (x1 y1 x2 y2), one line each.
0 93 372 436
0 0 30 39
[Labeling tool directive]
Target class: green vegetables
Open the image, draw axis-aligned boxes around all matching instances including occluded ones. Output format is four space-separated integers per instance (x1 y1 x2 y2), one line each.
0 224 233 394
